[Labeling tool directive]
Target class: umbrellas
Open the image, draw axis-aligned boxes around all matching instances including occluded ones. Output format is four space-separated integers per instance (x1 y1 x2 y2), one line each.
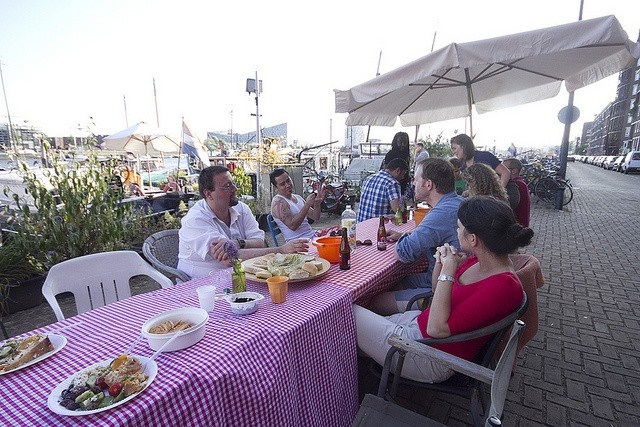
332 14 639 152
106 121 180 190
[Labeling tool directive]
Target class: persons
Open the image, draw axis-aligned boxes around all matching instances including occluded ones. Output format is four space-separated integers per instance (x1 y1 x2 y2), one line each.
270 169 325 244
450 133 520 212
176 166 309 285
461 163 510 205
352 195 535 383
414 143 429 176
507 141 518 158
378 131 409 195
357 158 409 222
502 158 530 228
394 158 473 316
448 158 468 195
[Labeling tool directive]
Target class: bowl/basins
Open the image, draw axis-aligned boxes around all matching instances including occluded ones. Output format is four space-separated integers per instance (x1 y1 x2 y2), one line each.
313 236 342 263
414 208 432 226
141 307 209 352
224 292 265 315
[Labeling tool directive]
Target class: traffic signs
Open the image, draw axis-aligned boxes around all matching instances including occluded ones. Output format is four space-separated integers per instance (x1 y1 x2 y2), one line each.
557 105 579 124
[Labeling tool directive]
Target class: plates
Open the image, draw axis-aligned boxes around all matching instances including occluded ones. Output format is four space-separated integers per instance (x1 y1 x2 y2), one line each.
240 253 331 282
48 355 159 416
0 332 68 375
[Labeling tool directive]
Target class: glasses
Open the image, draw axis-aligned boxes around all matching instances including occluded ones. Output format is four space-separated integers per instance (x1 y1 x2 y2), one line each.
211 181 238 189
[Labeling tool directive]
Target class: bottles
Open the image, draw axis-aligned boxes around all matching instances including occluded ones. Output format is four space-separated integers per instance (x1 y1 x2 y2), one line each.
395 207 403 225
342 205 357 253
401 201 407 223
378 215 386 249
339 227 351 271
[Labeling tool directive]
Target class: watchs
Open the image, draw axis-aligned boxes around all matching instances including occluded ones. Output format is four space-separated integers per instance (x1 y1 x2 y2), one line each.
437 274 454 283
237 239 246 249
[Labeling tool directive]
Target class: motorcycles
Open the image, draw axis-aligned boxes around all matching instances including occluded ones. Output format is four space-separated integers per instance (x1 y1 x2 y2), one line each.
306 178 349 223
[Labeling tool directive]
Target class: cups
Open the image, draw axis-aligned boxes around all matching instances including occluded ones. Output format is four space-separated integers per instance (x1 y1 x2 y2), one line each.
197 285 216 312
266 276 289 304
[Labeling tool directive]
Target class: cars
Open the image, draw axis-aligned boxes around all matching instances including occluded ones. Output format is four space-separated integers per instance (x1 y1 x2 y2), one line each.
566 156 574 161
568 154 606 166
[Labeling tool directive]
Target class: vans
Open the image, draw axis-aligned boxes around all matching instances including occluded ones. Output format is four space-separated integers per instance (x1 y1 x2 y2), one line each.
619 150 639 173
602 155 618 169
612 155 625 171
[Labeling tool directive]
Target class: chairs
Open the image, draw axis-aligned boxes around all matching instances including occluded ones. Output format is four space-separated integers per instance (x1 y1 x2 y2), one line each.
508 253 540 277
378 288 529 426
143 230 269 281
350 320 524 426
41 250 174 324
266 213 296 246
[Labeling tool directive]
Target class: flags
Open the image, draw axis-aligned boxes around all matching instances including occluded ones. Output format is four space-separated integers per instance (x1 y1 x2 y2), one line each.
181 114 209 166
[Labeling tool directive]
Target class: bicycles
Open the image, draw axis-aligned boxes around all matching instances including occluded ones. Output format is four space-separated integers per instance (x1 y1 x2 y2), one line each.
519 155 572 205
